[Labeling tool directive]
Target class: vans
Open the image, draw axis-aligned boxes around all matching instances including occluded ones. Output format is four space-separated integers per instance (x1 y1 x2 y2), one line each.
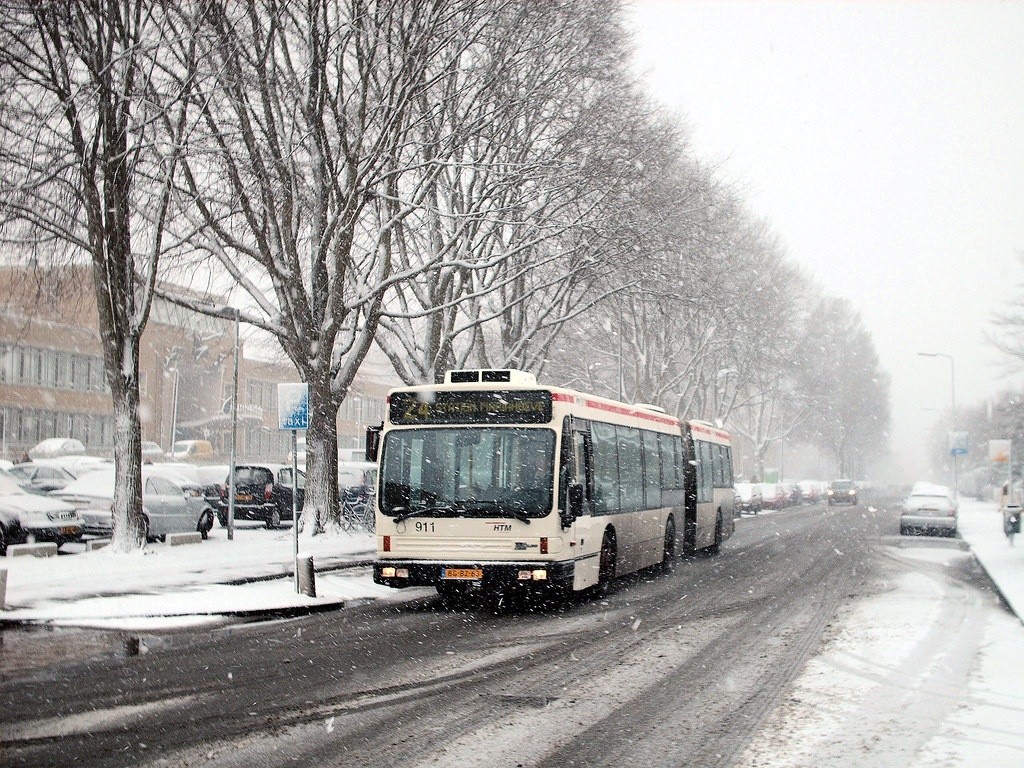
164 438 215 465
287 435 307 464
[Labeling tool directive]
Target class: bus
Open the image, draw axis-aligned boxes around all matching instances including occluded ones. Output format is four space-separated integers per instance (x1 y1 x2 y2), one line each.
362 367 738 610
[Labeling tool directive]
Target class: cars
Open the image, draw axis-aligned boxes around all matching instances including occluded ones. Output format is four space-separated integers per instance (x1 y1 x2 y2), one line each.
781 480 831 508
827 478 860 503
0 458 235 510
338 448 371 465
755 483 788 511
338 461 377 514
28 437 86 459
735 484 763 516
217 462 316 530
733 489 744 519
140 441 167 462
47 472 214 542
898 485 958 537
0 464 87 554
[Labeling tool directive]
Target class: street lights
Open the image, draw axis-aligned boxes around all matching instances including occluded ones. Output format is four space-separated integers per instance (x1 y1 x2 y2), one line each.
916 349 958 504
211 303 241 540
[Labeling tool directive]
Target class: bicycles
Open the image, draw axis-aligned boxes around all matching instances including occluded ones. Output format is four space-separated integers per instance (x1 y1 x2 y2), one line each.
334 485 377 532
